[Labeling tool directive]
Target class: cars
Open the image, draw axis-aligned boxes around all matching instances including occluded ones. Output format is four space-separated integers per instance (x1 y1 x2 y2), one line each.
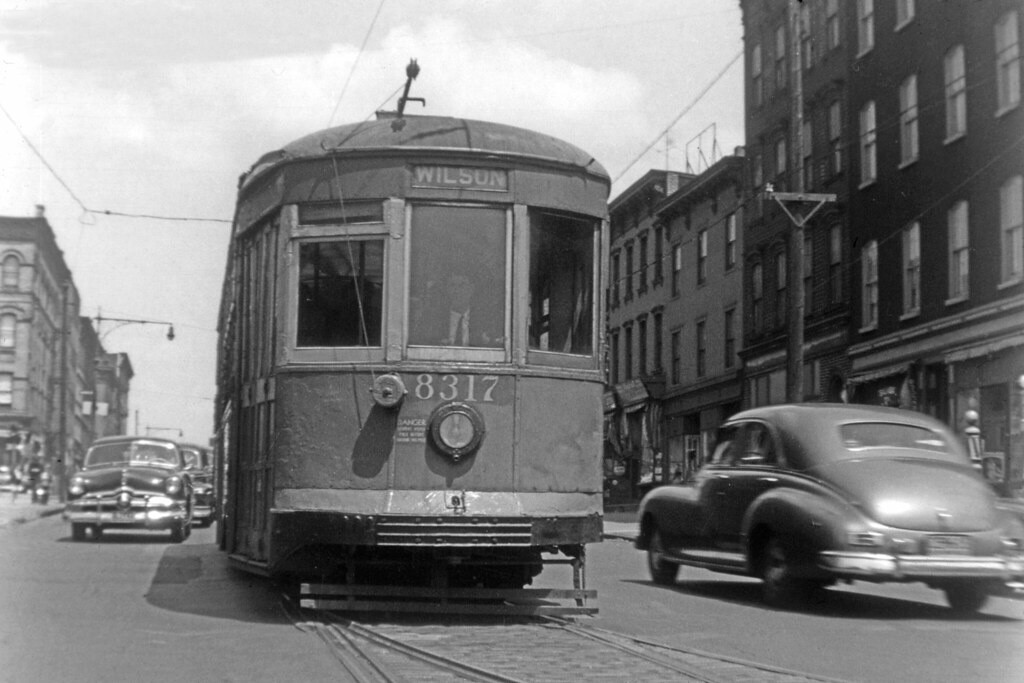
634 402 1024 616
64 436 193 542
179 444 213 525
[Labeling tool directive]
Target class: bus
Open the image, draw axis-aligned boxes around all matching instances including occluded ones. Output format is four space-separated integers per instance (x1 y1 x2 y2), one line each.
213 114 612 616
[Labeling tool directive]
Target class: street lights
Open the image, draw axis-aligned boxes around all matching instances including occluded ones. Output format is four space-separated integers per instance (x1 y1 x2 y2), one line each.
90 316 175 441
